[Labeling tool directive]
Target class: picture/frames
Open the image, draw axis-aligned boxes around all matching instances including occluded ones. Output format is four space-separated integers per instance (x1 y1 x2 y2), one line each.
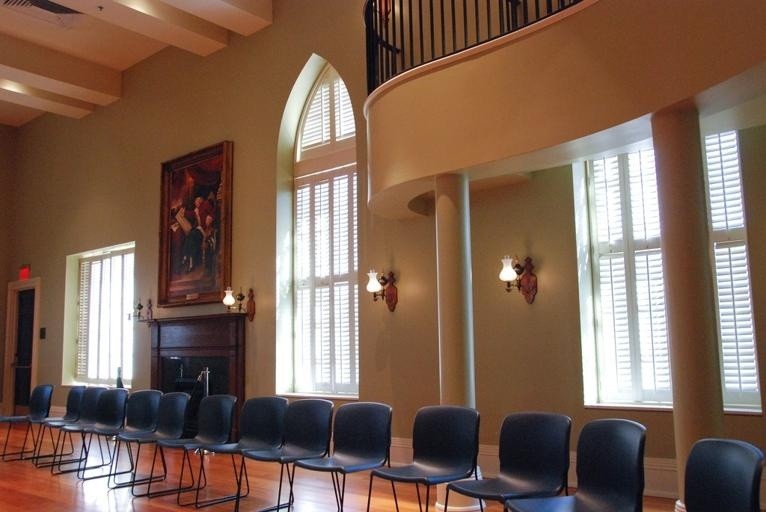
157 139 235 309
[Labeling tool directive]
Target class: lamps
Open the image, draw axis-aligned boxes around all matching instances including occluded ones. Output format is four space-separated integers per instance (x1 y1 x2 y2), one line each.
220 286 257 324
366 268 399 313
130 295 154 328
497 252 539 307
